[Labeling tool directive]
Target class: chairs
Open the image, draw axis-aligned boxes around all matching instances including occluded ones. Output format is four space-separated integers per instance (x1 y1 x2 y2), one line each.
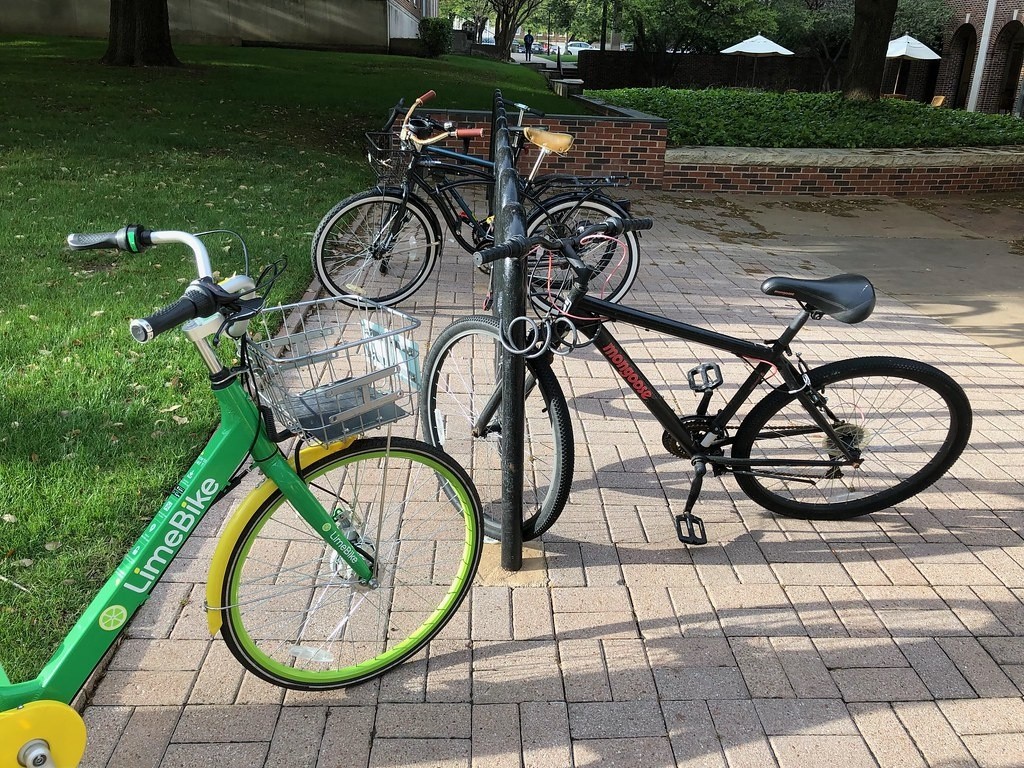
886 94 902 100
902 94 907 102
931 96 945 107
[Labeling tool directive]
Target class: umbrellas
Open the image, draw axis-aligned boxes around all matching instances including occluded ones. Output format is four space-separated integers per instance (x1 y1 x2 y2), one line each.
882 30 943 97
718 29 796 90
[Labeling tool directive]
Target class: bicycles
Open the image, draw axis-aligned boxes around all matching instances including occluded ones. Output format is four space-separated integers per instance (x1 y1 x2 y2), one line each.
311 89 641 320
0 225 486 768
378 97 619 289
418 217 975 547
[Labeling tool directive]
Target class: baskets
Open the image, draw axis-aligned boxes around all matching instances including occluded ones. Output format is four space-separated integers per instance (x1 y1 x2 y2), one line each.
365 130 414 184
235 292 425 451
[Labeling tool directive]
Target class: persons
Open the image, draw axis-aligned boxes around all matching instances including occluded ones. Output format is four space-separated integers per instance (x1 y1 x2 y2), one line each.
523 29 535 61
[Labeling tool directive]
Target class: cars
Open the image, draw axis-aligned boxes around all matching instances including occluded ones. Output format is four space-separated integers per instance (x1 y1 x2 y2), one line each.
565 41 600 55
510 40 558 54
